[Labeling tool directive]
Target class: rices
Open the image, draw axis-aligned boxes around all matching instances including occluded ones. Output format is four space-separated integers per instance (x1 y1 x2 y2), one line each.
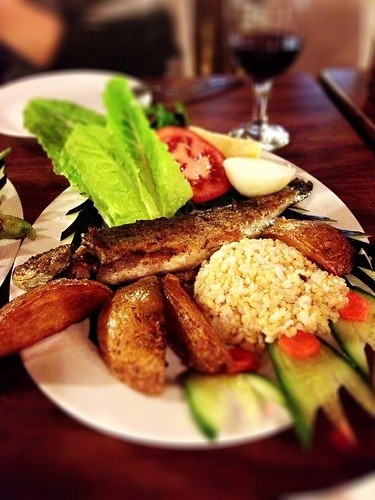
194 237 351 344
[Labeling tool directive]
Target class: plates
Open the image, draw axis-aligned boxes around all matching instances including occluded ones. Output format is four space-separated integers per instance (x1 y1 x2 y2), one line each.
1 70 153 139
9 147 372 449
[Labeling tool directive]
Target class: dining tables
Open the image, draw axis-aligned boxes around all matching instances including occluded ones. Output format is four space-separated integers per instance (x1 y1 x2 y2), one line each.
1 75 375 499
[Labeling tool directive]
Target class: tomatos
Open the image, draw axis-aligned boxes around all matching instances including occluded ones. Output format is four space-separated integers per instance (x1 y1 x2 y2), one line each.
155 124 232 205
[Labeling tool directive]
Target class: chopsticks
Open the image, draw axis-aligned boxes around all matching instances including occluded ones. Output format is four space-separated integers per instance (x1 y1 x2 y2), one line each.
0 170 24 290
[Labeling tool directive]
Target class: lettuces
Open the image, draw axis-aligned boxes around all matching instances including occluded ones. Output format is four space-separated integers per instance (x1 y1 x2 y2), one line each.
22 77 193 230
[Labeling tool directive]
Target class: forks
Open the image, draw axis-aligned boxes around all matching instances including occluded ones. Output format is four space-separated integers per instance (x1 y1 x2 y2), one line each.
131 76 241 98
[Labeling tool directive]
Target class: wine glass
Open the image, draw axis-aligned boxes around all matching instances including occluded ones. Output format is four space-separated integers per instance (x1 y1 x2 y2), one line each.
225 1 312 152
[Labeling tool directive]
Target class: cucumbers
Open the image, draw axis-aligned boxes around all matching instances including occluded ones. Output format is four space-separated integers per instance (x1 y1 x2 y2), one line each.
184 288 374 450
